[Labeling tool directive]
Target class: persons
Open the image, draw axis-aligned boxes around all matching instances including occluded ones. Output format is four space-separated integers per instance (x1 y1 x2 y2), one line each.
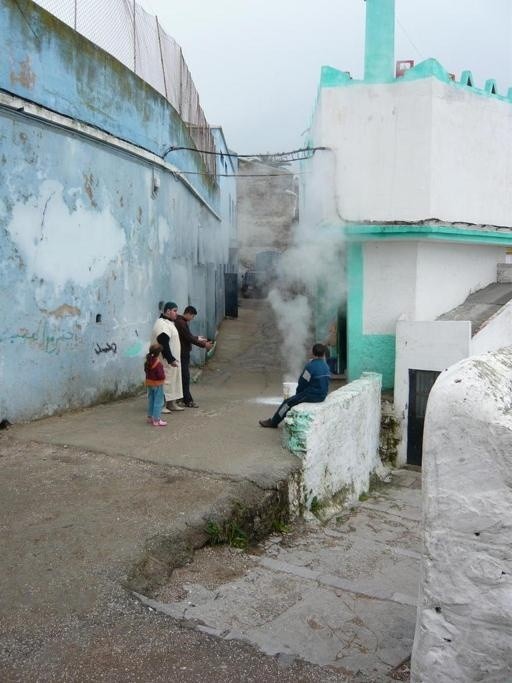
259 343 332 428
151 301 185 415
144 344 169 425
175 306 215 409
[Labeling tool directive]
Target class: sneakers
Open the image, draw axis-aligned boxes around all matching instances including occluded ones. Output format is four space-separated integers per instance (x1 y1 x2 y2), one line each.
186 401 199 408
161 406 170 414
146 418 152 422
260 419 279 429
154 419 166 427
167 405 184 411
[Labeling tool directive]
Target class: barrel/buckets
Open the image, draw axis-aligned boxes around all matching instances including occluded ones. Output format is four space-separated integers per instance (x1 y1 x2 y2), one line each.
282 382 299 400
